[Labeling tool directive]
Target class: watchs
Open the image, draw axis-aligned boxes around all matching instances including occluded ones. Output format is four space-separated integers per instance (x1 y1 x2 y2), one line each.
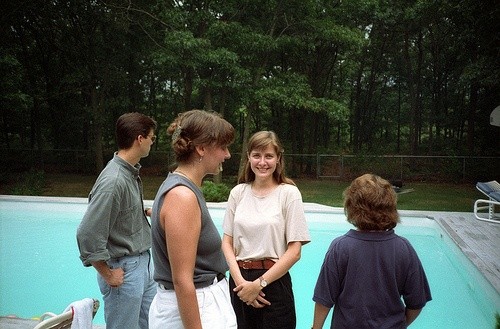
259 276 268 287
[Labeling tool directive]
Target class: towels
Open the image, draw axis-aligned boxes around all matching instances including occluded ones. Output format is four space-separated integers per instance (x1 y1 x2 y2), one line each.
62 297 95 329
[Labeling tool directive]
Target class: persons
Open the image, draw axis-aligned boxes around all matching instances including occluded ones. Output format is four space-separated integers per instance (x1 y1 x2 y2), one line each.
148 110 238 329
75 112 152 329
311 174 433 329
222 131 311 329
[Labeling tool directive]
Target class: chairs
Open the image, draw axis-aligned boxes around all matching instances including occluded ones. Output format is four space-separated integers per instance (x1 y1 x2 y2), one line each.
0 299 99 329
474 180 500 224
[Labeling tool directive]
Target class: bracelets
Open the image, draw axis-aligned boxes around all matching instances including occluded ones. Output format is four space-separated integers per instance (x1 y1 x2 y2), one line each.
145 208 150 215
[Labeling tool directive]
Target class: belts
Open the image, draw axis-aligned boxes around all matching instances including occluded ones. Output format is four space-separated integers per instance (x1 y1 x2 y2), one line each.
164 272 226 291
237 260 275 269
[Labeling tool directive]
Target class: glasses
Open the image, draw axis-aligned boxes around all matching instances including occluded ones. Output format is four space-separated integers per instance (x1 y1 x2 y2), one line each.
148 135 156 142
